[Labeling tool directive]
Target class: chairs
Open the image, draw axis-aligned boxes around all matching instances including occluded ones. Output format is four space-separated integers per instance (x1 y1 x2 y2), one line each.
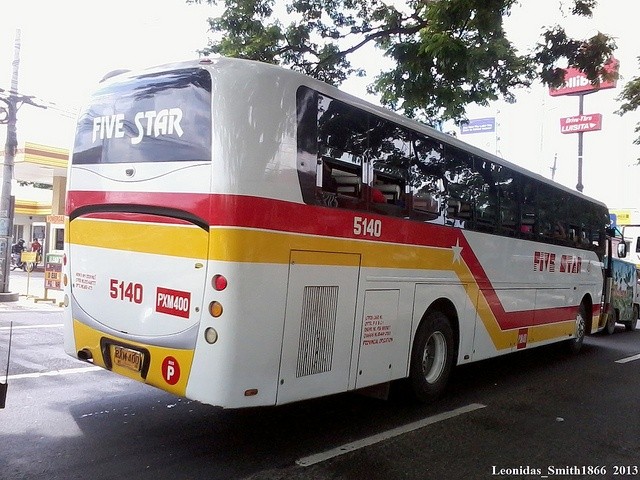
373 183 401 205
413 194 433 211
334 177 361 198
569 229 579 240
583 231 585 239
448 199 462 215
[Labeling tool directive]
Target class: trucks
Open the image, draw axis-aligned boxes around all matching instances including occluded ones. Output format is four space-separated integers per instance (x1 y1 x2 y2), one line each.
604 255 640 334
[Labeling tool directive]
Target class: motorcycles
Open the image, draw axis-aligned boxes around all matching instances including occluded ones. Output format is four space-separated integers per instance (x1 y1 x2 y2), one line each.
10 247 34 272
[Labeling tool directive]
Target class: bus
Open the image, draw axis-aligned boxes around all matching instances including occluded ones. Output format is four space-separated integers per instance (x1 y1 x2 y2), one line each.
62 57 626 409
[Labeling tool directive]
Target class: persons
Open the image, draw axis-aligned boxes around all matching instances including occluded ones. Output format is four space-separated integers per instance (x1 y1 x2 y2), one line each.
12 238 26 267
29 237 42 253
553 221 566 241
